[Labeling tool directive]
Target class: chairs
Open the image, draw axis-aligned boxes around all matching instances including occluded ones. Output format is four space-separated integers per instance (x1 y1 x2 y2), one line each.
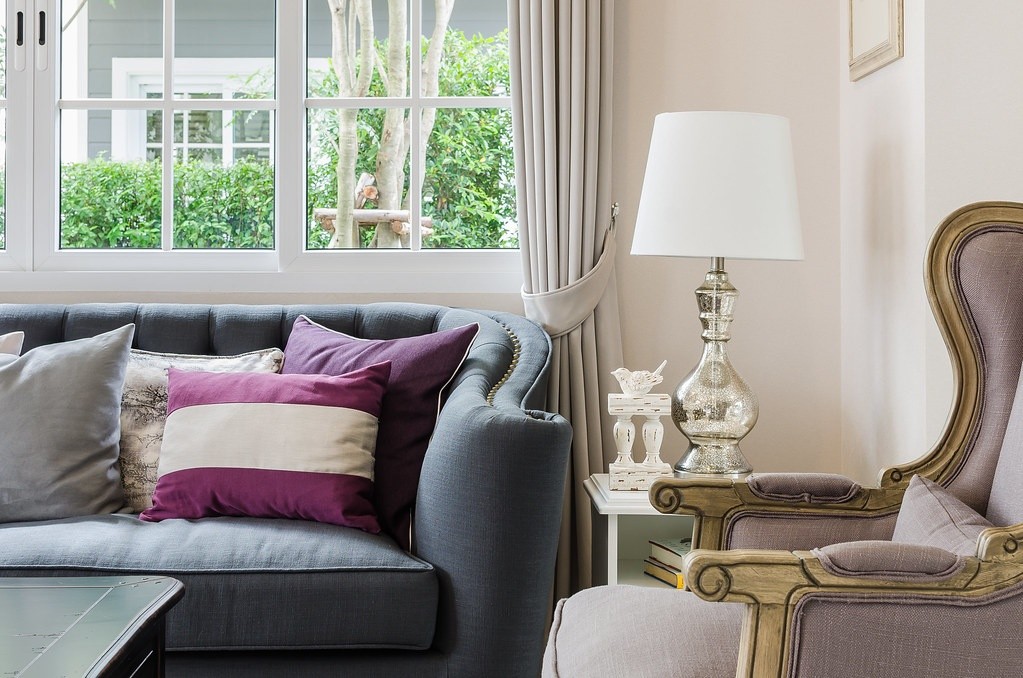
539 200 1022 678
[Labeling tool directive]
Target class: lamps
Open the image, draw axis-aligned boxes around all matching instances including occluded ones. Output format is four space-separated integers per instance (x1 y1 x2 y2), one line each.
627 111 805 479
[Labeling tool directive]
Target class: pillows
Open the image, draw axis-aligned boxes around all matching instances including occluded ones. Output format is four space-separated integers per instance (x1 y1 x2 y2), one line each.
892 470 995 556
119 347 283 514
280 313 481 555
137 361 392 535
0 323 136 521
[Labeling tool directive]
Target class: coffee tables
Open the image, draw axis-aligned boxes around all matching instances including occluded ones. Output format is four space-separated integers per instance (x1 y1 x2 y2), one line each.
0 575 187 678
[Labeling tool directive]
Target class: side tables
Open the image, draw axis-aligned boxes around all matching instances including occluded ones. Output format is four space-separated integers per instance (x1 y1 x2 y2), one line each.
581 473 778 586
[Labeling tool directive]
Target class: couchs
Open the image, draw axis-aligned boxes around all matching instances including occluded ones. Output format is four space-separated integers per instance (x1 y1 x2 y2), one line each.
0 304 572 678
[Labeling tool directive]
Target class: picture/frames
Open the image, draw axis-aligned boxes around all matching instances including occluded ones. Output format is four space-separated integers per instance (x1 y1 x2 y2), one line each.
849 0 903 83
